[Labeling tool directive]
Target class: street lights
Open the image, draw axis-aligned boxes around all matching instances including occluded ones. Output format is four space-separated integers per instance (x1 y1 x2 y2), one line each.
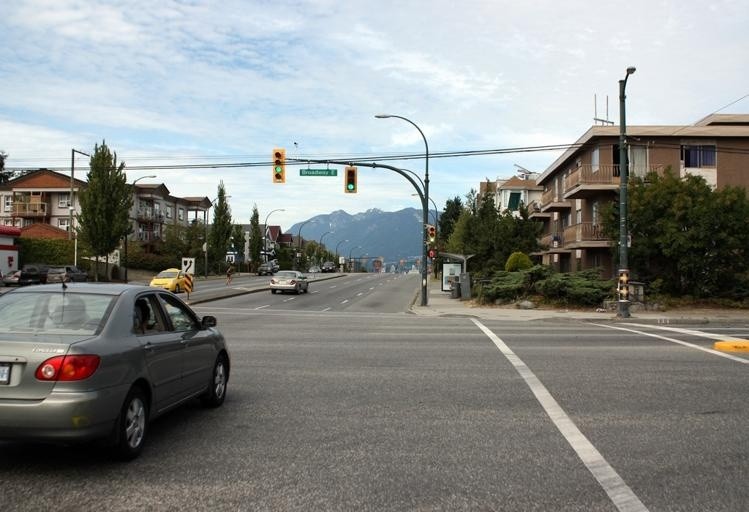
125 176 156 283
374 115 428 308
618 66 637 319
204 195 364 275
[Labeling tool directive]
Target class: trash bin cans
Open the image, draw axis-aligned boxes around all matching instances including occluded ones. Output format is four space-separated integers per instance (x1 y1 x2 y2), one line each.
450 282 461 298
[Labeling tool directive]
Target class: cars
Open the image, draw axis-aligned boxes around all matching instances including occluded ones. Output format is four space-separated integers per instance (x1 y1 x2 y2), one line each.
0 276 232 464
322 261 336 272
149 267 193 293
269 270 308 295
0 262 127 284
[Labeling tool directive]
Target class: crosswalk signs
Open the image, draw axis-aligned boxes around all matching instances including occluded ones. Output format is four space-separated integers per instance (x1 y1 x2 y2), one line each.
428 250 437 259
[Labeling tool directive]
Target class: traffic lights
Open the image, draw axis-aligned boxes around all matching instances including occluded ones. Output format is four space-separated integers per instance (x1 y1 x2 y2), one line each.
428 225 437 243
273 149 285 183
344 166 358 194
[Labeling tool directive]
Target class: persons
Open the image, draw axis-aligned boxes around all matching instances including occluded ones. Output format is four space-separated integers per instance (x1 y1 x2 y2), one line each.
67 297 91 329
135 297 159 335
225 265 235 287
